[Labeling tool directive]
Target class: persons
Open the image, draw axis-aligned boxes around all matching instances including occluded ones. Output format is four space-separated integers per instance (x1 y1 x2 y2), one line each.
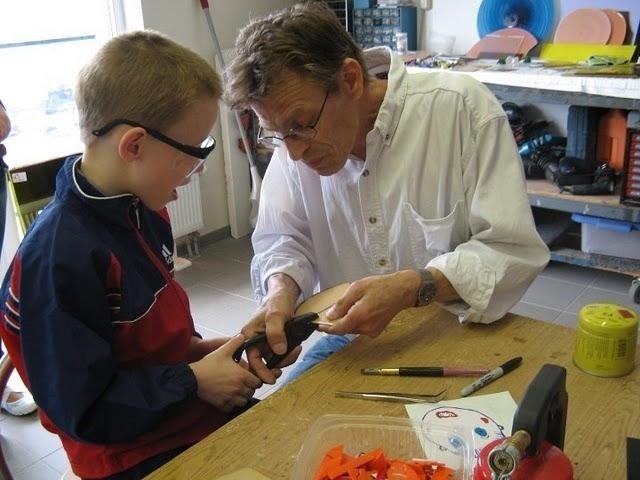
0 101 40 416
220 3 552 400
0 31 263 478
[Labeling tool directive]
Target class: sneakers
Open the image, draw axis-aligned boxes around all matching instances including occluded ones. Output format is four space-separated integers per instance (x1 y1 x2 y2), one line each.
0 385 38 417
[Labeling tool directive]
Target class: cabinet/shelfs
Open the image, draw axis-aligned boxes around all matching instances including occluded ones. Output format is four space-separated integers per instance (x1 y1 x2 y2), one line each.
455 64 640 281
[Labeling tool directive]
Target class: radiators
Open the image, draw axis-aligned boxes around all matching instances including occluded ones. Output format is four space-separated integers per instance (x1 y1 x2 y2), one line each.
165 170 207 261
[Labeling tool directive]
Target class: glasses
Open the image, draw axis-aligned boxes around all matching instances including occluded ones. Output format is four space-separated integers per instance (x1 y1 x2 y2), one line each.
92 119 216 178
257 91 330 149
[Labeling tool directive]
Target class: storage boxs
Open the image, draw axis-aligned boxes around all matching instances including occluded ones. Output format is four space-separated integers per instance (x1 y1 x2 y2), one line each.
572 208 639 258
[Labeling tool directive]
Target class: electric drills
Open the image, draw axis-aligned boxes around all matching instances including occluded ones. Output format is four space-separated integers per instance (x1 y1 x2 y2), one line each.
559 163 615 196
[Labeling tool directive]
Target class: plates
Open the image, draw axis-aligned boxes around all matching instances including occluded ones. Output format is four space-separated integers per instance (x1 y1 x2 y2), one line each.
552 7 628 45
477 0 556 45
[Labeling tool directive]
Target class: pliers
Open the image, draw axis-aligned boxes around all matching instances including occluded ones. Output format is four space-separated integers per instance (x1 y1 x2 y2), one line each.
232 312 320 383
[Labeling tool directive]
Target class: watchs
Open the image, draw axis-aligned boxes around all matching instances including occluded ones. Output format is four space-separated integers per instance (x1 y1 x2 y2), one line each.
414 265 438 306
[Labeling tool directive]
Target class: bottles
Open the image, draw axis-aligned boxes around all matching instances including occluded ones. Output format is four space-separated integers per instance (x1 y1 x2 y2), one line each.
395 32 408 54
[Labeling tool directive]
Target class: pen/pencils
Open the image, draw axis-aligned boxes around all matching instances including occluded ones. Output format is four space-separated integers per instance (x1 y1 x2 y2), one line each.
459 356 523 397
360 367 490 376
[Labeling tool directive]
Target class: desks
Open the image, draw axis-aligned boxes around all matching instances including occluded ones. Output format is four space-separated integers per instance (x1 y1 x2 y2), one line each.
133 292 640 479
